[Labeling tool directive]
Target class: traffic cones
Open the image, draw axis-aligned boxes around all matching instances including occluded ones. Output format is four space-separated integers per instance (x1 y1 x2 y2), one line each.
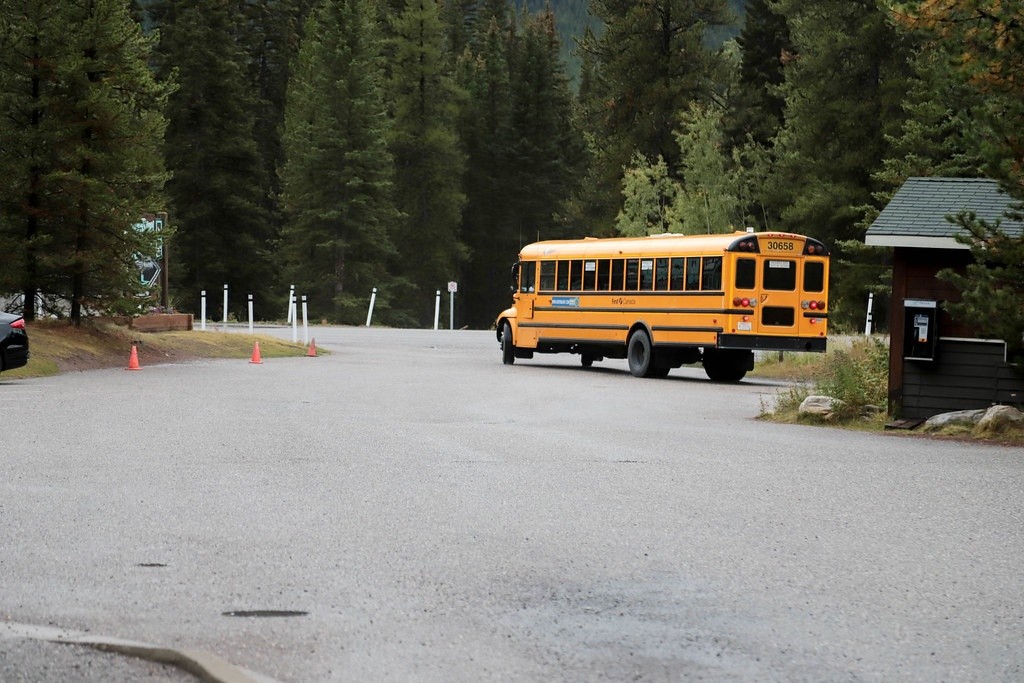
249 341 264 365
124 345 142 371
304 337 320 357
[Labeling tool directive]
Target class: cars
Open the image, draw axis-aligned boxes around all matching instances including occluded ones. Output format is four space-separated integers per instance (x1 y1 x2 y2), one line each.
0 312 30 375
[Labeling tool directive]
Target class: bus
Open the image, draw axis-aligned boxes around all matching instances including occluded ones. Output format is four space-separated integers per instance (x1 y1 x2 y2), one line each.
496 225 832 386
